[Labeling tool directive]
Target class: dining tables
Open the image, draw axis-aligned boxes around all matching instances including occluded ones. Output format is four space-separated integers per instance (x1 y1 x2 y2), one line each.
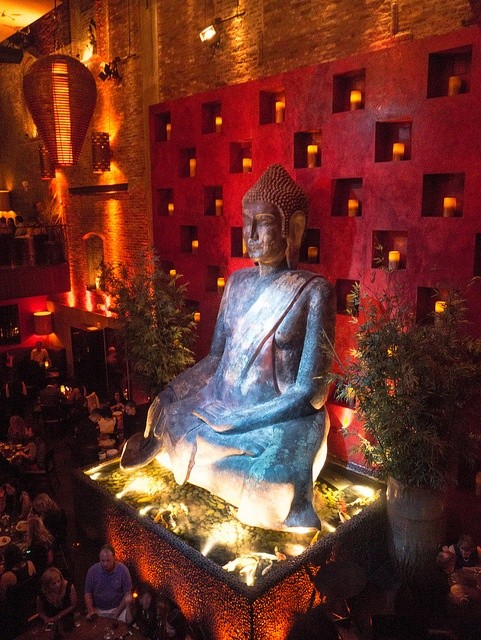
0 515 26 561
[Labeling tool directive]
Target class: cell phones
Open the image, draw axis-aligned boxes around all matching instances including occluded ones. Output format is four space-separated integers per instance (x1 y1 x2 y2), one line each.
45 622 54 631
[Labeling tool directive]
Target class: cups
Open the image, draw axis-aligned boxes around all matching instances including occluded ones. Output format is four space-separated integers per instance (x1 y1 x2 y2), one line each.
74 612 81 627
103 627 111 640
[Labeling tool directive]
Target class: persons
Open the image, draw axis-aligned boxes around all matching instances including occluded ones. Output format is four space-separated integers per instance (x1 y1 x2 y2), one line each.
36 567 78 628
128 587 192 640
0 477 67 607
0 216 27 235
120 166 336 533
0 415 52 475
336 535 481 640
0 342 147 465
84 544 132 622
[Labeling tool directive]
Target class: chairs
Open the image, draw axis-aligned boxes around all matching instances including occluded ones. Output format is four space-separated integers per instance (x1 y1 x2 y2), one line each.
22 447 61 493
40 404 66 440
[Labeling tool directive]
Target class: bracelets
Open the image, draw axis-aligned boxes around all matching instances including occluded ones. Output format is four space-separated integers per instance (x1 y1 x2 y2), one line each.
57 614 62 619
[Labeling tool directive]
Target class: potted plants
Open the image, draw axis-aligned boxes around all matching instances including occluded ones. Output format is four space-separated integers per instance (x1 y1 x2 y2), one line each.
311 236 481 595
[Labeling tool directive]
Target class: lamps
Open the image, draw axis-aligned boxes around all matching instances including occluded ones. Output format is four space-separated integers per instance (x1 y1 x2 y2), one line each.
98 53 137 81
199 13 245 42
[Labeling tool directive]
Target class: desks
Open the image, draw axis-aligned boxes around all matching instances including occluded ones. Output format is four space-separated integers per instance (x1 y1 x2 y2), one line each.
15 614 146 640
0 441 28 463
